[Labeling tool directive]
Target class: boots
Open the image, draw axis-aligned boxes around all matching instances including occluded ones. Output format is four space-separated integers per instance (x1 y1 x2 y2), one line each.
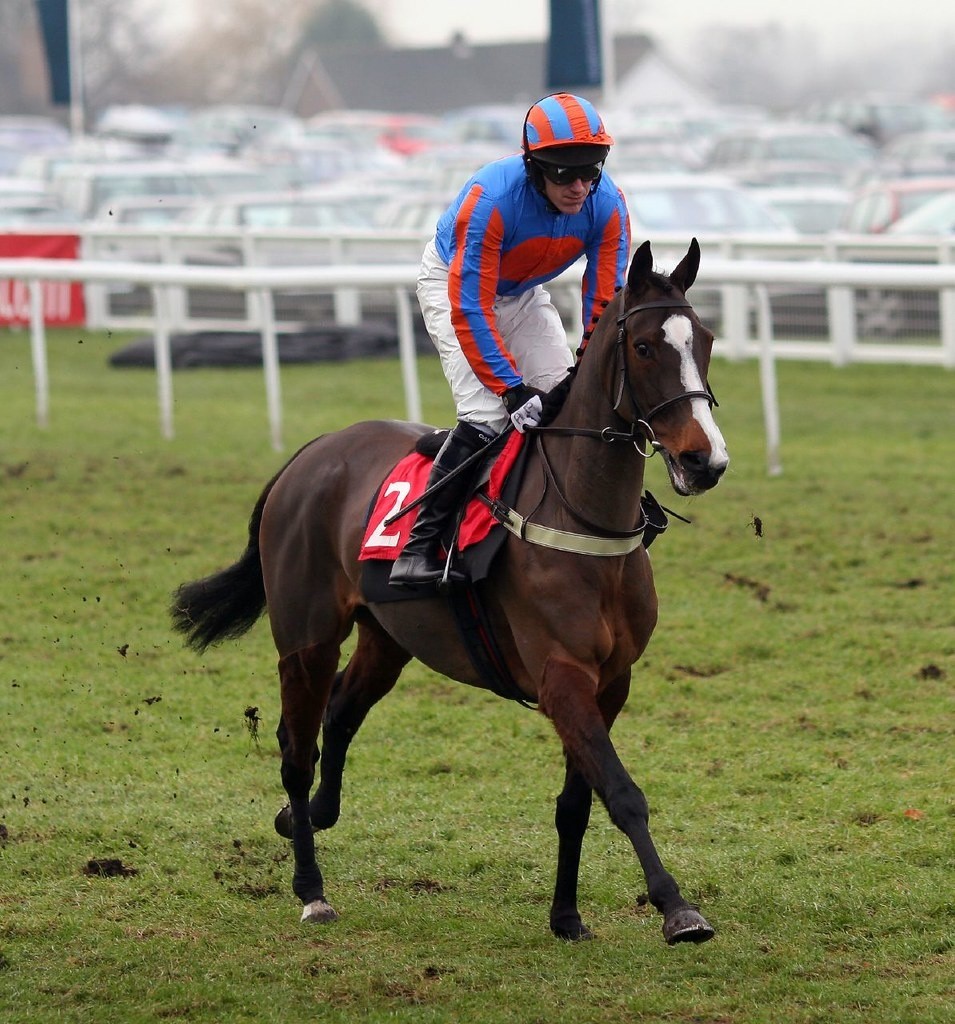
386 430 490 593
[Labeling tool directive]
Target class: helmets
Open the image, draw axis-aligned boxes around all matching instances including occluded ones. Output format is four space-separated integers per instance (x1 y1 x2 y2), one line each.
520 144 612 161
522 92 614 152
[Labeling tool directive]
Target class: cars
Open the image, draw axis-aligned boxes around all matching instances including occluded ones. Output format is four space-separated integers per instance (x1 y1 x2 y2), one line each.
0 110 955 236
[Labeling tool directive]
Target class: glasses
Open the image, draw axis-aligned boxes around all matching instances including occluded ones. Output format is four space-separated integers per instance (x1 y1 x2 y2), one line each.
530 154 604 184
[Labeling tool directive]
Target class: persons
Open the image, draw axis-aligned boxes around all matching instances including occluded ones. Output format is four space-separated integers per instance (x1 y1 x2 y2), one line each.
386 91 631 585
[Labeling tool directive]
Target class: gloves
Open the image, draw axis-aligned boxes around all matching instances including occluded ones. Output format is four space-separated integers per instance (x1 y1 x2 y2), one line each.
502 383 556 433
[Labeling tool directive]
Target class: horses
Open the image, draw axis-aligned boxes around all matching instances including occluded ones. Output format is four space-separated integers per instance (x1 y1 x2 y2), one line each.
164 237 731 946
